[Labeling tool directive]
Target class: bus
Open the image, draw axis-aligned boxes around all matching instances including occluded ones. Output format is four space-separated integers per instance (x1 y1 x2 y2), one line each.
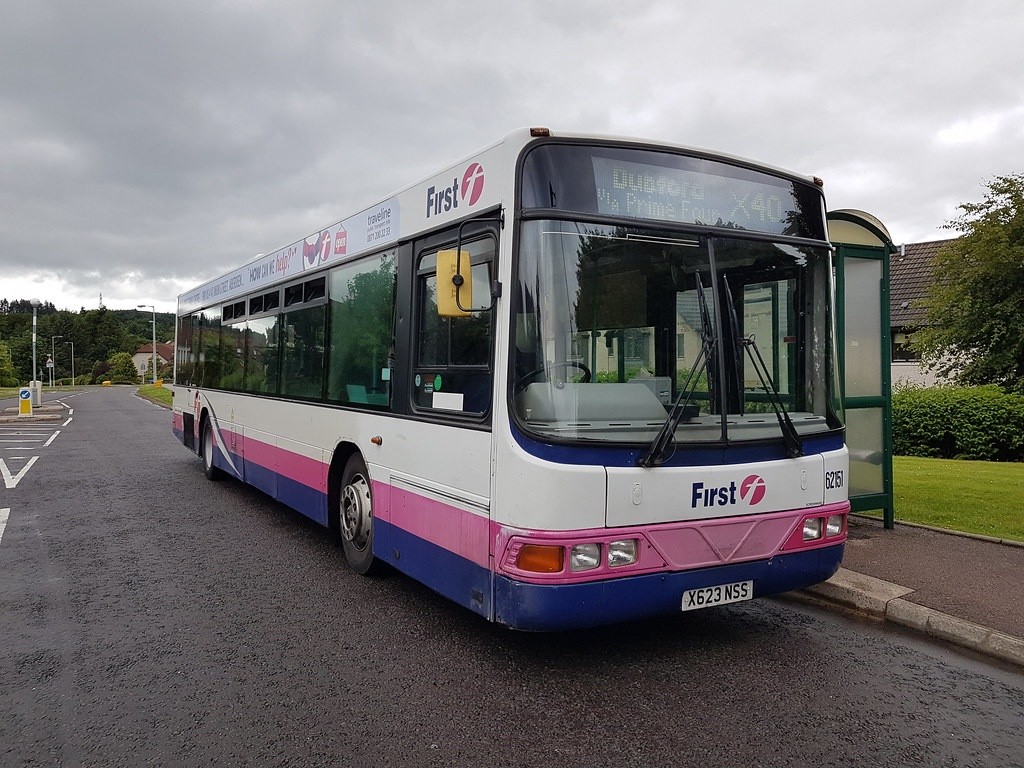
171 126 852 635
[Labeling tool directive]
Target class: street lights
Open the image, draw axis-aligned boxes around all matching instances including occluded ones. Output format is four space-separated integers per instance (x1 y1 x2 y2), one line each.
138 305 158 386
52 335 63 389
65 341 75 387
